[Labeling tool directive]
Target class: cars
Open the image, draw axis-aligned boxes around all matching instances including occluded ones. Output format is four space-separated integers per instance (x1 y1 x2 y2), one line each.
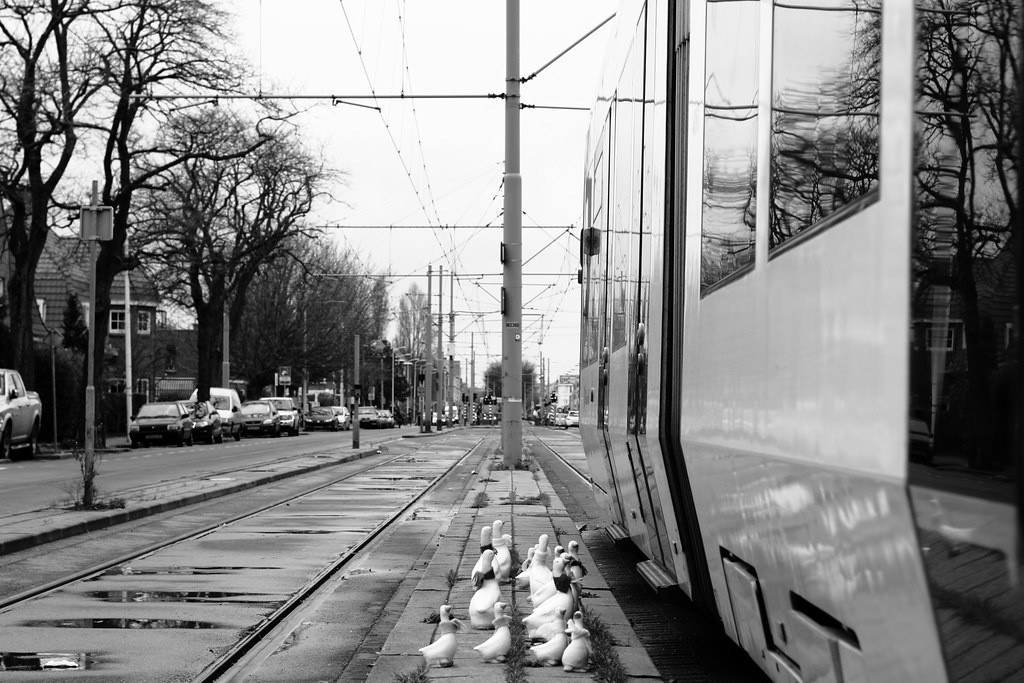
304 406 339 432
331 406 350 431
353 406 395 430
566 410 579 426
240 400 281 438
129 402 195 449
260 396 300 436
173 399 223 444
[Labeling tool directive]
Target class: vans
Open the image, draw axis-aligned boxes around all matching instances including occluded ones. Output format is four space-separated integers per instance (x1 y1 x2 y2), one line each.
189 387 242 441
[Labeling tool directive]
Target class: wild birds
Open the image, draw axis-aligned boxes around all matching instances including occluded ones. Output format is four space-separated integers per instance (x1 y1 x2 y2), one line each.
418 520 593 673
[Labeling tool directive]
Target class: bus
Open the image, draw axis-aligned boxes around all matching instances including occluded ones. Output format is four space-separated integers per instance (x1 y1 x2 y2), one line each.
575 3 1024 683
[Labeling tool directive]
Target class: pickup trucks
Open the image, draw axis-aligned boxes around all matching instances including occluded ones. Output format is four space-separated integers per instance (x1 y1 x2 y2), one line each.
0 368 42 458
